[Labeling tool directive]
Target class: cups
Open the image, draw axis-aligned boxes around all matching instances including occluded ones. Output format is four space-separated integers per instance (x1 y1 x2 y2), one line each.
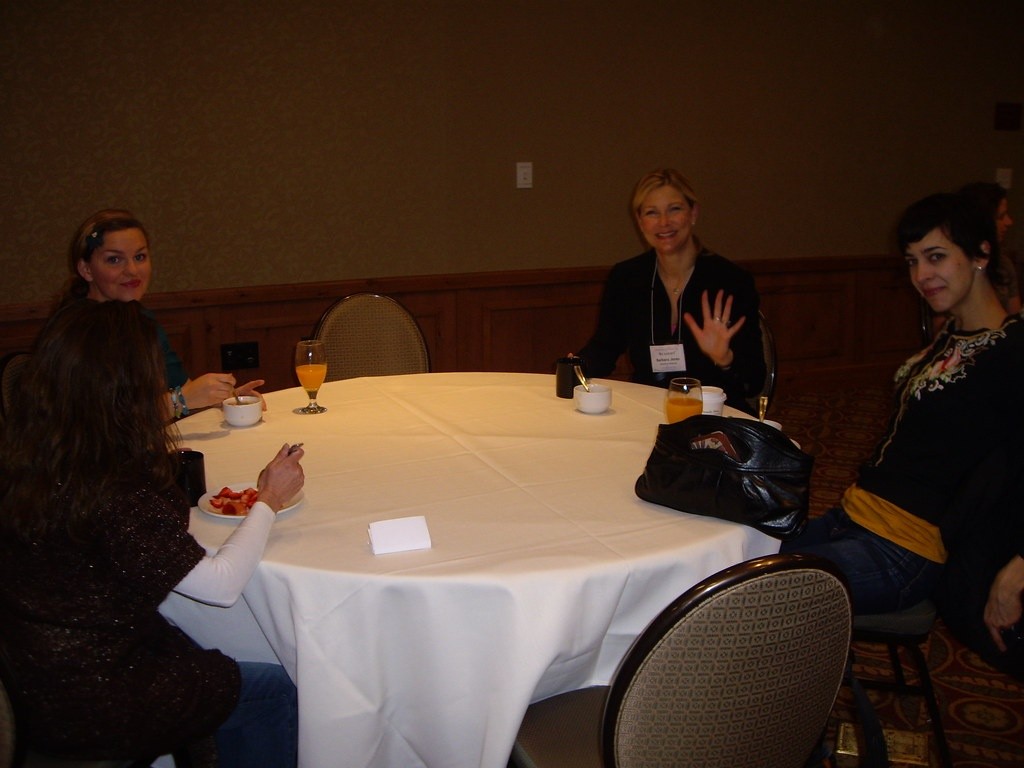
556 357 582 399
754 419 781 431
790 439 800 450
665 377 704 422
700 386 726 418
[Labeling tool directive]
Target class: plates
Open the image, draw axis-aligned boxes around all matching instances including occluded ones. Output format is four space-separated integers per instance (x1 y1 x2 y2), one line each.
198 481 305 519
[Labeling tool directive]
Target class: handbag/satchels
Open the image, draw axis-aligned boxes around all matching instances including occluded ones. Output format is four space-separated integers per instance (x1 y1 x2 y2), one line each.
635 414 815 542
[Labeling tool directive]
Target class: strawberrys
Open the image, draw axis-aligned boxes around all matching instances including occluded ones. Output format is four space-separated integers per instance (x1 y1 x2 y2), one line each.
210 487 260 516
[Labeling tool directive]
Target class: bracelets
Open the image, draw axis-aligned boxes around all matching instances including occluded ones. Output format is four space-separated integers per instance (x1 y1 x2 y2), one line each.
168 385 188 419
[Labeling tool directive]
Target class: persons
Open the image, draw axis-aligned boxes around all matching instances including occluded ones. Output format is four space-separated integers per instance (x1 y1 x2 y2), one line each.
779 190 1024 768
0 301 305 768
930 182 1020 342
42 208 267 426
565 167 766 432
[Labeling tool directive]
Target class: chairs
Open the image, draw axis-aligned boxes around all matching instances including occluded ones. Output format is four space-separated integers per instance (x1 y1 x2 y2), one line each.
845 600 955 768
308 291 433 383
0 351 33 422
745 310 777 415
0 681 160 768
508 552 853 767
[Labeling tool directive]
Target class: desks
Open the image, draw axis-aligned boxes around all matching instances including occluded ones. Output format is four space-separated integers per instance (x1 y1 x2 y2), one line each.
154 373 803 768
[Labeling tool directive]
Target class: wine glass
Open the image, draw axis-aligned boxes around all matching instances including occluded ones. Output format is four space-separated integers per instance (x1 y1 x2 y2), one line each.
295 340 327 414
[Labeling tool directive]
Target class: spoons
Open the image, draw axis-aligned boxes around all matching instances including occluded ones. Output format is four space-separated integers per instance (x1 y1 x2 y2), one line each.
223 396 263 426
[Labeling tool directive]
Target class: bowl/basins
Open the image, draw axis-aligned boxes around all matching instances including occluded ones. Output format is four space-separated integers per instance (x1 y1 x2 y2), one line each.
573 384 612 415
231 390 248 405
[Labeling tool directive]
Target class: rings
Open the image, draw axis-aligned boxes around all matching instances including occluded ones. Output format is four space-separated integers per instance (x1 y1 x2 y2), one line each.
714 316 722 321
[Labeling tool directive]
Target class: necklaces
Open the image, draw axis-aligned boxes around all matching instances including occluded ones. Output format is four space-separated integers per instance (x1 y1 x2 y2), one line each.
659 263 693 295
